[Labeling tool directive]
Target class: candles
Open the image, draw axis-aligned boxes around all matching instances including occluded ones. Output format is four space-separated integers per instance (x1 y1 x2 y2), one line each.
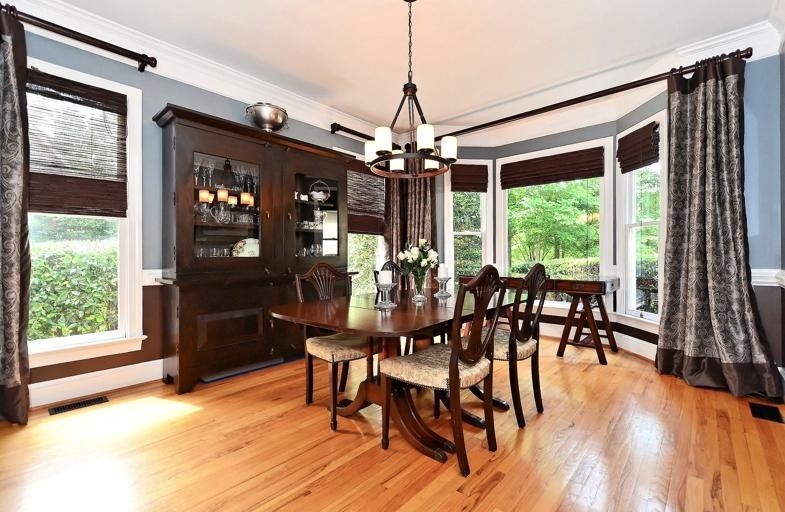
197 188 256 210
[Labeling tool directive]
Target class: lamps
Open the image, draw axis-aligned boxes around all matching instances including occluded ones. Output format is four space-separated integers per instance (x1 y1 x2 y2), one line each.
357 0 460 181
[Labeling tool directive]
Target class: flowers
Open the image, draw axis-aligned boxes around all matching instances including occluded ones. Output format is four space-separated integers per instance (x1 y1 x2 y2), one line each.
397 236 442 295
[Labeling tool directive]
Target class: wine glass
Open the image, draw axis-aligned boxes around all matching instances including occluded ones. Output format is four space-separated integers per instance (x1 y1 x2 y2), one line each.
231 163 259 195
193 154 216 187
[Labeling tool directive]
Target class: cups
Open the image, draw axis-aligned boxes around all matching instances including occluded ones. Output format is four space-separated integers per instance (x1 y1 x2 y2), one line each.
296 243 322 257
295 220 314 229
197 200 255 225
195 247 230 257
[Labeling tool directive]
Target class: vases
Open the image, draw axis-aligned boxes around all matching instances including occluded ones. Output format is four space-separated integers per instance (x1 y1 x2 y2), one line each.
411 274 429 303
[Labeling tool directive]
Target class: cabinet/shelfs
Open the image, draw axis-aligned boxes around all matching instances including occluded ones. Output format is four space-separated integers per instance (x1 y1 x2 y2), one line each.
151 101 357 281
153 269 360 396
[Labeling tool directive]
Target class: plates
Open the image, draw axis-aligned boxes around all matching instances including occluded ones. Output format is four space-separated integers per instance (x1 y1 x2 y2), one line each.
231 237 259 258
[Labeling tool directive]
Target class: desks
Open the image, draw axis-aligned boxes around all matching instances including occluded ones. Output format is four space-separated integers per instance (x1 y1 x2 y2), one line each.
456 271 621 367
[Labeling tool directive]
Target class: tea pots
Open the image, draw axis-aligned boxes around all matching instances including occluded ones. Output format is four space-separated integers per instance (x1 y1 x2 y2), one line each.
312 206 327 229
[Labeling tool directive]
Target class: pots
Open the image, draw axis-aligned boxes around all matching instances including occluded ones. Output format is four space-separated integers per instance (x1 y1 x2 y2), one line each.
246 103 289 131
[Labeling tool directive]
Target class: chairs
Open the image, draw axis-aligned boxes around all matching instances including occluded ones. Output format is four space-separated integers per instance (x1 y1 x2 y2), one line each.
293 261 382 434
442 259 549 429
371 258 426 399
376 264 508 479
